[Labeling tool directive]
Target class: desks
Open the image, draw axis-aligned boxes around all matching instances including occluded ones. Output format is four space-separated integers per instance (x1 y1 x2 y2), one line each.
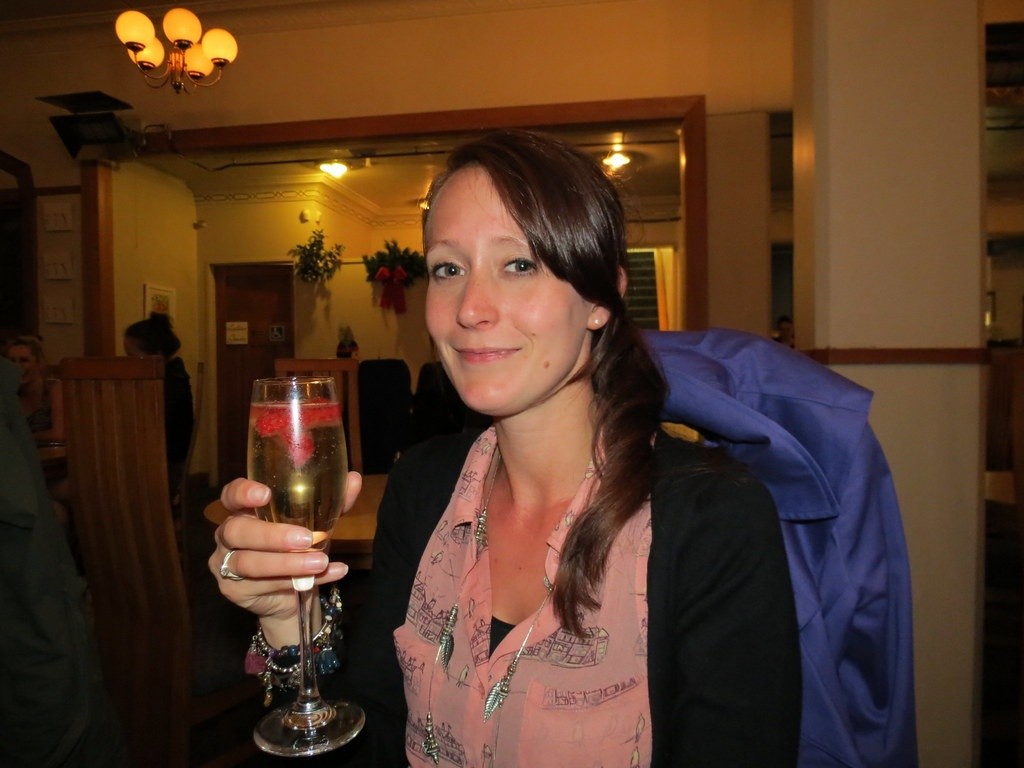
327 472 390 571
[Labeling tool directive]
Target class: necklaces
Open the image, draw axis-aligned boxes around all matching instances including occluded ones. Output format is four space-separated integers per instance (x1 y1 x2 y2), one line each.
421 450 594 768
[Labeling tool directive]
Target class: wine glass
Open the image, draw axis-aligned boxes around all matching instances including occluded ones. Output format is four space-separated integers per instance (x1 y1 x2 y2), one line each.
246 375 366 757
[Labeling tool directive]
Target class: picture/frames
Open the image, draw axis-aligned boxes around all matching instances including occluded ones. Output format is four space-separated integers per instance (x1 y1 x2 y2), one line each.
142 282 177 329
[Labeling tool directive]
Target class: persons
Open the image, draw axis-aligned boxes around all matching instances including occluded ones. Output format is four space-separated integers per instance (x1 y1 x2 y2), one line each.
1 303 196 768
208 129 802 768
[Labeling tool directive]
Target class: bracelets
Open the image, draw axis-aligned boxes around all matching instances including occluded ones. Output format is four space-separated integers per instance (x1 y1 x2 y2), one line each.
248 586 345 708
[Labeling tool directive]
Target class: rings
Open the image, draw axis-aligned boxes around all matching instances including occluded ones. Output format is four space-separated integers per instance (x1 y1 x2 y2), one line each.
220 549 244 581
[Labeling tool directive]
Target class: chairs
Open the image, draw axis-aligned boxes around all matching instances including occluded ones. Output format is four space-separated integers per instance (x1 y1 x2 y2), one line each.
273 358 363 476
58 352 269 768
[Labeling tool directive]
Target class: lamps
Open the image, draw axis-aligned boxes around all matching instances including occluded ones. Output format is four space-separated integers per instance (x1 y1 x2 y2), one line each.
115 0 239 95
